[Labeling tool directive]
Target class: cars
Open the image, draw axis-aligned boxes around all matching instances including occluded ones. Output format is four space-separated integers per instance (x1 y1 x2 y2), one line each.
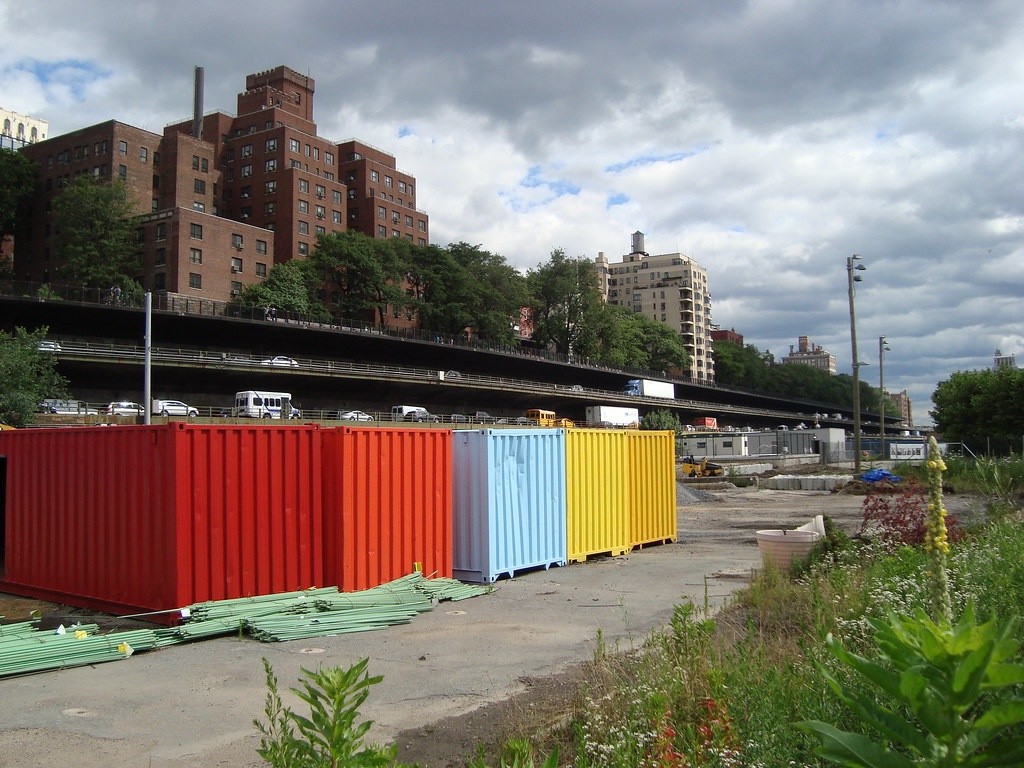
259 354 301 368
159 400 200 418
443 411 533 427
341 410 374 421
404 409 440 423
682 409 929 439
26 340 62 352
107 402 145 416
554 417 576 428
443 369 462 379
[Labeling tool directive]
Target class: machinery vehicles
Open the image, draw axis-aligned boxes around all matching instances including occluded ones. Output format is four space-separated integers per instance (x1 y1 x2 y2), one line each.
683 455 723 477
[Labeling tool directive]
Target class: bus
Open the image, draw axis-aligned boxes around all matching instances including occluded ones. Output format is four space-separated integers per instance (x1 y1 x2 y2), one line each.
525 409 556 427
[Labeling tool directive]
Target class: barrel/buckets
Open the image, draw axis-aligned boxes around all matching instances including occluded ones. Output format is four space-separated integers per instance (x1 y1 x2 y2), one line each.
755 529 820 573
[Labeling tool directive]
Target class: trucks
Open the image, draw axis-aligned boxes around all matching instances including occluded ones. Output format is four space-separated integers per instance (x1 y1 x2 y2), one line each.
622 379 675 400
586 405 644 429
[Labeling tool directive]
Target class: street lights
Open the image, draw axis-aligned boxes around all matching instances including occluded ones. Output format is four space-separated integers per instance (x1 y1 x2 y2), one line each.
847 253 871 475
879 335 892 460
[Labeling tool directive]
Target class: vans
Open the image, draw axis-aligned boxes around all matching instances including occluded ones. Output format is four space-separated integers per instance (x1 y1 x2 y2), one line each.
235 390 301 420
43 398 100 415
391 405 429 421
